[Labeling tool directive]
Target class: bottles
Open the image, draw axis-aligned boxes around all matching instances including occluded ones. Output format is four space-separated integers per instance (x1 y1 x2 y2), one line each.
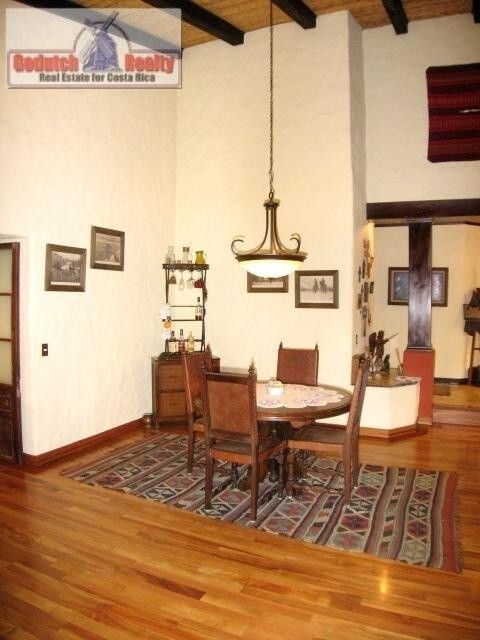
169 328 195 355
165 246 193 264
194 298 202 321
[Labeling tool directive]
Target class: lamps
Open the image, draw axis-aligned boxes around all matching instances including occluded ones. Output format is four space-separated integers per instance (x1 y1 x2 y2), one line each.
228 1 307 279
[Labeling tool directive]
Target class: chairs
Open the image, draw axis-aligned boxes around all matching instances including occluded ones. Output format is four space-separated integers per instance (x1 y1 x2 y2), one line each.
282 358 371 504
200 359 287 521
274 342 319 390
182 347 254 490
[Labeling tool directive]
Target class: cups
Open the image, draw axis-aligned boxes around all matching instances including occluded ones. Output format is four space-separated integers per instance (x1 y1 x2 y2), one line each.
210 357 221 373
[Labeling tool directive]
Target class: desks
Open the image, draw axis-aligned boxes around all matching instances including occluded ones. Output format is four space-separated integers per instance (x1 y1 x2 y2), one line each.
194 382 353 491
352 366 424 441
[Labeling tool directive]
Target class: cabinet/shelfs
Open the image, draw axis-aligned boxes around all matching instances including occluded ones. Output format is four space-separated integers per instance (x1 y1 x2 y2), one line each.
151 351 222 429
164 263 209 350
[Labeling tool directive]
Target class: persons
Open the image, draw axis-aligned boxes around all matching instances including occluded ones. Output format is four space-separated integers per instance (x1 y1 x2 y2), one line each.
312 278 319 292
320 278 328 293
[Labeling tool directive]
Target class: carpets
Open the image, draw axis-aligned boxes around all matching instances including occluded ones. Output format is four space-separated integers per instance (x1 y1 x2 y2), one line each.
59 431 465 579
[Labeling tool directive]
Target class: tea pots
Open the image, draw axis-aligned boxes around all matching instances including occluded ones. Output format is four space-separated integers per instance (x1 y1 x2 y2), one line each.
194 251 209 266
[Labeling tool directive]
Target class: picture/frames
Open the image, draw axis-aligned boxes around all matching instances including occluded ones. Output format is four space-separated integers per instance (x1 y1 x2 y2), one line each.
247 270 290 294
293 268 340 309
88 226 125 271
387 267 449 308
43 243 88 292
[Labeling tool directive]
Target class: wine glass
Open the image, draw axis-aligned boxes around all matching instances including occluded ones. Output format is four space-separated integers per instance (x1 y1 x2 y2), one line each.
169 270 205 291
